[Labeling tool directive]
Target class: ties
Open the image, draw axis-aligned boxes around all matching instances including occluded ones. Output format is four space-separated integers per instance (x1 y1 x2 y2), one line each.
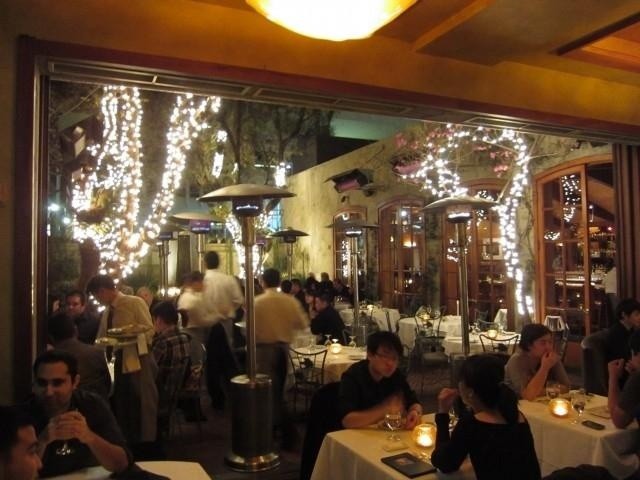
106 306 114 362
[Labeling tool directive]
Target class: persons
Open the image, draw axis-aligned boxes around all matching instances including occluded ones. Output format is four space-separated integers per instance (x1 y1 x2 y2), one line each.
431 353 542 479
144 300 191 400
301 267 380 293
255 269 310 452
280 280 291 291
46 313 112 398
605 297 640 393
25 350 135 474
393 261 437 314
504 323 570 402
607 329 640 480
63 290 100 343
175 269 227 422
0 401 45 480
310 291 349 345
291 279 305 309
330 332 423 433
601 263 620 326
551 253 565 308
477 281 501 319
85 276 160 461
564 222 582 266
303 290 319 320
86 279 163 311
201 251 245 411
49 292 61 314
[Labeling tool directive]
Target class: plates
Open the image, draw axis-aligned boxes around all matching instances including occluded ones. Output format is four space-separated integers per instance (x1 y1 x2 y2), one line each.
108 324 150 334
93 337 117 346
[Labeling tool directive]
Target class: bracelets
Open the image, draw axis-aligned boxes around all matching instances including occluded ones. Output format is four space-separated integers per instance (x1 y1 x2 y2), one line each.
407 408 420 416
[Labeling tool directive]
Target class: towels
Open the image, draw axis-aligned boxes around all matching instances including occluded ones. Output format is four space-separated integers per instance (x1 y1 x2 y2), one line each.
121 334 148 375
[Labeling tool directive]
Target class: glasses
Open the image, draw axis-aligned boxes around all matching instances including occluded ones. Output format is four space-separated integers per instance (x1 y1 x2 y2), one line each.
374 352 397 361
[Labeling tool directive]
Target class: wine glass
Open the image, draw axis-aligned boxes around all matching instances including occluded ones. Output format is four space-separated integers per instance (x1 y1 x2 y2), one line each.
385 407 400 441
474 322 479 329
324 335 332 346
569 389 586 425
547 381 560 398
56 408 77 455
348 336 356 348
471 326 477 336
